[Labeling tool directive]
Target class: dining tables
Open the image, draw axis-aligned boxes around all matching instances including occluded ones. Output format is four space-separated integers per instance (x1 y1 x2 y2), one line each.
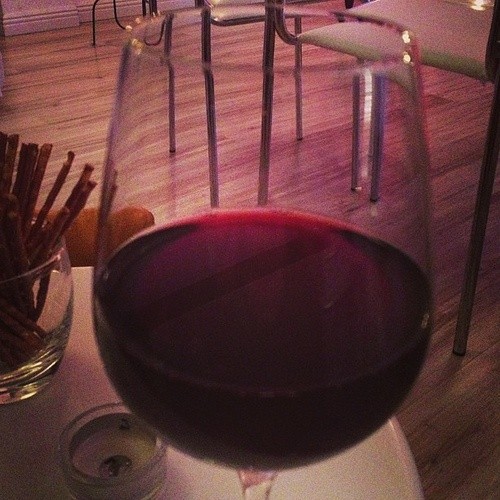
1 266 423 500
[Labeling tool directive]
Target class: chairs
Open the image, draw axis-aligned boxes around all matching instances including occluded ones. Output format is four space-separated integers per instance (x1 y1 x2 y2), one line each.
149 1 500 359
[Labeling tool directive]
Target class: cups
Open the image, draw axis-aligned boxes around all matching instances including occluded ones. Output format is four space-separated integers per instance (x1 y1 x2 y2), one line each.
0 219 75 405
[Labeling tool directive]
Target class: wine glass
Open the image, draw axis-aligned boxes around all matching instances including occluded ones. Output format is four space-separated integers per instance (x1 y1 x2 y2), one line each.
87 6 434 500
